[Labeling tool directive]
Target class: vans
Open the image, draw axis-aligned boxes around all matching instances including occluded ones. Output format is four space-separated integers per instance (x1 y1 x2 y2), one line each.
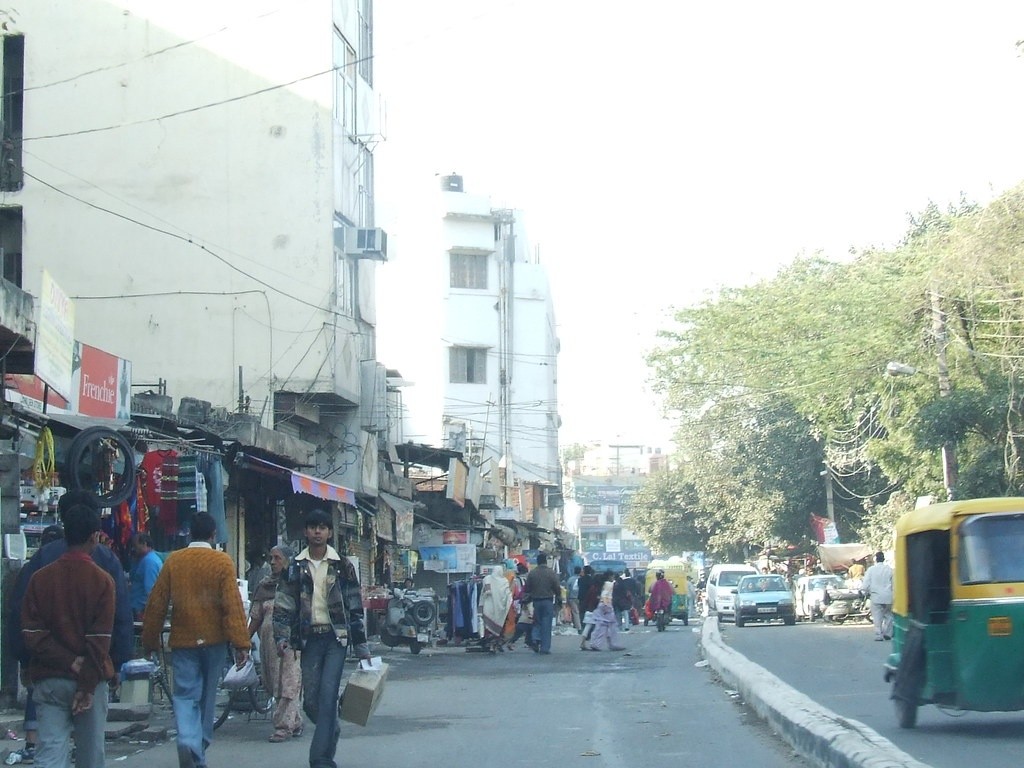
795 575 843 622
705 564 761 619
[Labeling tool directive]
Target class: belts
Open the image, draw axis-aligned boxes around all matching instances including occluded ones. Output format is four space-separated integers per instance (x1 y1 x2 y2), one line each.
308 624 333 634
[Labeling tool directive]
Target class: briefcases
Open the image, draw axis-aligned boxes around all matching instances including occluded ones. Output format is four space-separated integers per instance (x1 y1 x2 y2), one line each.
338 660 389 726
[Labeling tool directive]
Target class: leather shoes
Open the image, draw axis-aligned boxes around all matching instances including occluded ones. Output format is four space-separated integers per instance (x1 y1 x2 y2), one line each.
293 724 303 737
270 731 290 742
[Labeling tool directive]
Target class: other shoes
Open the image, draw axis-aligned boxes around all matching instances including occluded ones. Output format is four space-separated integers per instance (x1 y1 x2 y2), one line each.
875 637 883 641
884 635 891 640
507 644 514 650
497 645 504 652
539 651 551 655
532 641 539 653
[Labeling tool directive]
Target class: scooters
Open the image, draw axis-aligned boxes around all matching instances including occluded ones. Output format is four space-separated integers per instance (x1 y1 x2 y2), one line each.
382 577 436 654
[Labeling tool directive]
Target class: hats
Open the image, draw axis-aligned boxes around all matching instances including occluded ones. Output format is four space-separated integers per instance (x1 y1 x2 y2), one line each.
43 525 64 541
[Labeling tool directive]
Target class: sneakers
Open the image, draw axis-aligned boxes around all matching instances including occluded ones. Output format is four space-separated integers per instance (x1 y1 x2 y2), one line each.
10 748 34 764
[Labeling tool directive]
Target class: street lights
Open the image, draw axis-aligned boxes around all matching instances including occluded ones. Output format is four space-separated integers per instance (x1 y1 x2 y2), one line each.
887 362 958 502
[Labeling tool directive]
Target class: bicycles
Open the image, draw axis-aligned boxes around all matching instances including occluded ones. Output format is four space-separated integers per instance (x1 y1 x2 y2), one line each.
212 642 277 725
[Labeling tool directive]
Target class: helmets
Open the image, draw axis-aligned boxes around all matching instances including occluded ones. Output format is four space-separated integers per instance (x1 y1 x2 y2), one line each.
656 569 664 579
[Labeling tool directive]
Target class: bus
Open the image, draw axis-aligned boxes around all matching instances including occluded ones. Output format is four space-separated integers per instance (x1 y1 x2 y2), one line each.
590 560 627 576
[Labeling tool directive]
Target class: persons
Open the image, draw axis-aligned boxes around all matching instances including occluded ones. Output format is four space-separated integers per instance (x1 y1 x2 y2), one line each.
761 562 825 621
9 490 132 768
479 554 708 655
847 551 893 641
747 582 756 592
272 512 373 768
131 511 252 768
245 545 303 743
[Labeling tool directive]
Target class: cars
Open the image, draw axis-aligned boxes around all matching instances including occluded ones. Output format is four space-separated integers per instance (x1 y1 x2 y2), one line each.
732 573 795 628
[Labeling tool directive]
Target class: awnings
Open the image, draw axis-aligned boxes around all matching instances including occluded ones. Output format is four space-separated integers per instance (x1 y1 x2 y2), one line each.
233 452 357 508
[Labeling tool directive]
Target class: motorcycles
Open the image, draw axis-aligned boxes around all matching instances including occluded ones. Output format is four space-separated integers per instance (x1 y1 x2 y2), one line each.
642 562 689 625
823 586 875 625
655 585 677 632
881 496 1024 729
